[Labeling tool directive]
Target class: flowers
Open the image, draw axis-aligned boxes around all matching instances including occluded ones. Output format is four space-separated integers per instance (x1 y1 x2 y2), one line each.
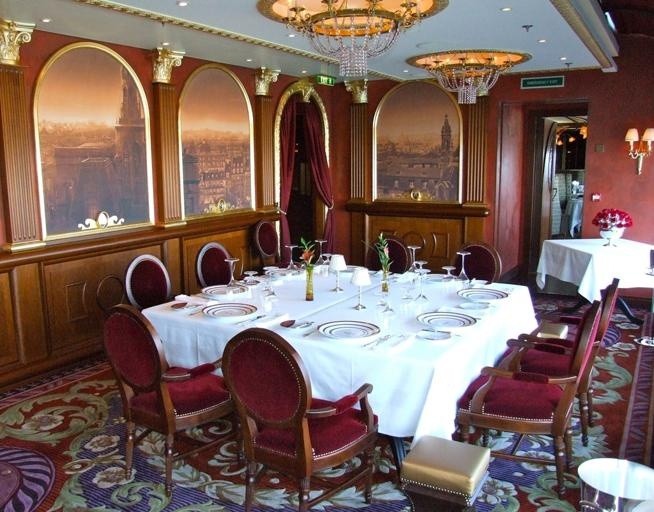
299 237 315 265
374 233 393 273
592 209 633 231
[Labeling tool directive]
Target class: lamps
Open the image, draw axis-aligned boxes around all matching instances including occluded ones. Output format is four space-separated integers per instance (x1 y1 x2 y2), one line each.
625 128 654 176
257 0 450 77
406 49 532 104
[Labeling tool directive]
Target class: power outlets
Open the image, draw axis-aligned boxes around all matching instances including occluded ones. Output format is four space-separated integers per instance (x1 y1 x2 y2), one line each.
591 192 600 203
594 143 604 153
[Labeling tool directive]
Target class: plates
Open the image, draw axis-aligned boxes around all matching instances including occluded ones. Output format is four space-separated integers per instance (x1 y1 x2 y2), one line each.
202 285 248 296
457 288 509 300
416 312 477 328
319 321 380 339
203 302 257 321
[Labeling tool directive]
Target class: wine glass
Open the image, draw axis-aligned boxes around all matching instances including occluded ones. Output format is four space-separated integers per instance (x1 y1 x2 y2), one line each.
415 261 428 269
413 269 431 314
287 245 300 286
457 251 470 289
225 258 242 301
441 266 457 293
244 271 258 298
262 266 280 316
315 239 328 281
323 253 332 277
406 246 421 286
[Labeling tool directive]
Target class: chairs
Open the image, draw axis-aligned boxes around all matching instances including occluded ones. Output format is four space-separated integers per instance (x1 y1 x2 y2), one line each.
124 253 171 311
101 304 244 499
366 235 411 274
254 219 291 268
503 278 619 446
195 241 233 288
451 241 503 283
456 301 603 500
221 327 378 512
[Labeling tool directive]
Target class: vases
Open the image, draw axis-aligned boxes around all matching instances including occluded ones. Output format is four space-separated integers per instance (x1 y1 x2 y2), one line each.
382 272 388 296
305 264 314 301
600 228 624 247
